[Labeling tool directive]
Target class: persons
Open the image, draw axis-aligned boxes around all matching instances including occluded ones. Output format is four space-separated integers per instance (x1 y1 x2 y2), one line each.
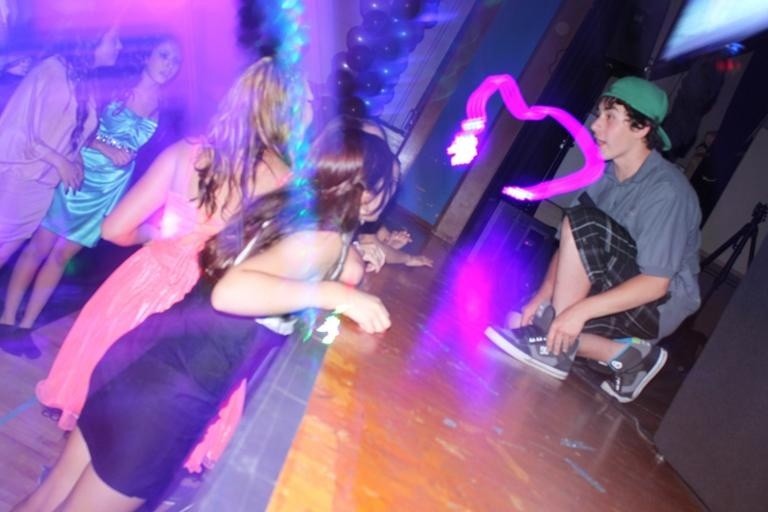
1 1 438 512
483 76 704 405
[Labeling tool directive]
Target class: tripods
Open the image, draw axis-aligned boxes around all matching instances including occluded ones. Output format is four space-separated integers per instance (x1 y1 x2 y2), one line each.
699 200 768 319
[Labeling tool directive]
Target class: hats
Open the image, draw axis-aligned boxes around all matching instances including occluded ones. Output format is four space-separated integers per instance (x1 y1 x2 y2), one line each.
603 77 672 151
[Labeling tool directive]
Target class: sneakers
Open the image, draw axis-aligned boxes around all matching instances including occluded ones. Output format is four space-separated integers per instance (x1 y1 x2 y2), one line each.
1 323 40 359
485 302 580 381
600 335 667 405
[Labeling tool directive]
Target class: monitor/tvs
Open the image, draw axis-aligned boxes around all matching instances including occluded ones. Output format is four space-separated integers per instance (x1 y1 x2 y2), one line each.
644 0 768 79
